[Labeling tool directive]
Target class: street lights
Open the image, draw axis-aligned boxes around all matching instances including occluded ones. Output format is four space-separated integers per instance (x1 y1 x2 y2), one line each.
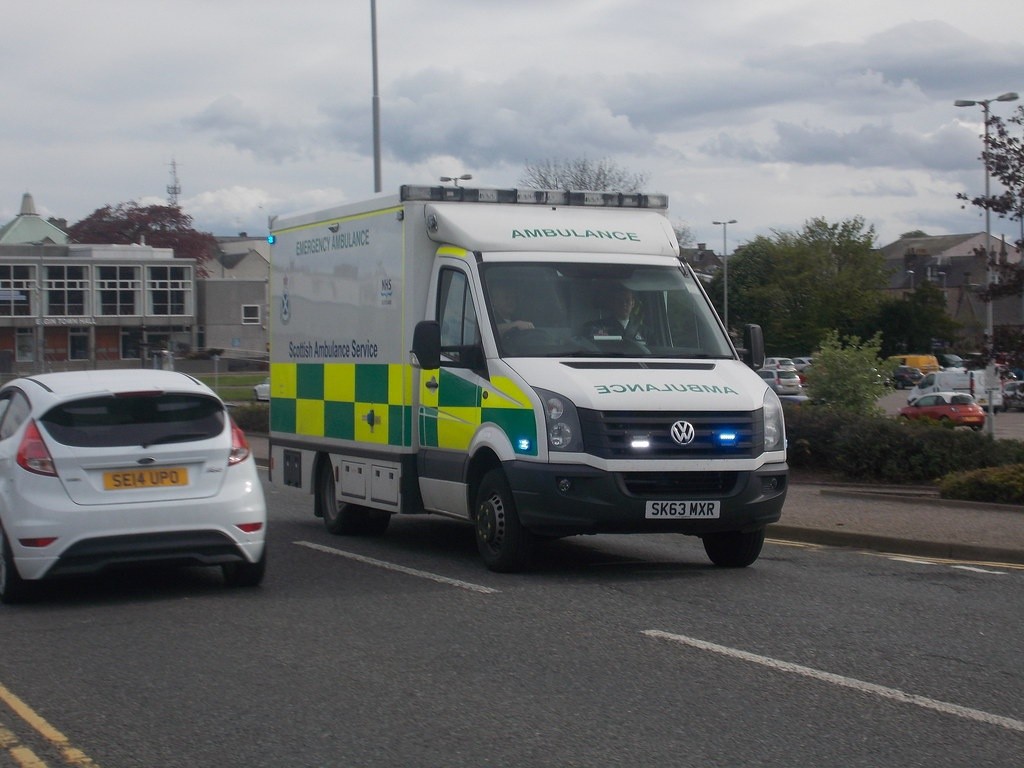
440 174 472 186
712 220 737 332
955 93 1020 360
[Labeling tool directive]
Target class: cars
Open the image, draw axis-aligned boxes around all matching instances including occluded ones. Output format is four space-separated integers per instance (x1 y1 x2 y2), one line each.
792 357 815 372
894 366 925 390
896 391 985 431
937 354 964 369
764 357 796 371
1001 381 1024 410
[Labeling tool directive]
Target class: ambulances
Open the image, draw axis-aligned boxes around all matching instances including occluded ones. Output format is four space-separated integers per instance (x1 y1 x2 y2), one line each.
268 185 790 573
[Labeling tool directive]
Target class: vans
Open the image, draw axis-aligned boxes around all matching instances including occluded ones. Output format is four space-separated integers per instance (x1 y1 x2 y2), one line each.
906 368 1003 416
888 355 939 374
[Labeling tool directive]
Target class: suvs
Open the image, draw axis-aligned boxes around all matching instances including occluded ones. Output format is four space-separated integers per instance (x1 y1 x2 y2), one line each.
0 368 267 605
755 369 801 395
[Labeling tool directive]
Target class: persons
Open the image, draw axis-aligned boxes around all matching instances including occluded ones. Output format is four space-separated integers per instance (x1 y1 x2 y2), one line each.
486 286 535 341
584 285 655 347
